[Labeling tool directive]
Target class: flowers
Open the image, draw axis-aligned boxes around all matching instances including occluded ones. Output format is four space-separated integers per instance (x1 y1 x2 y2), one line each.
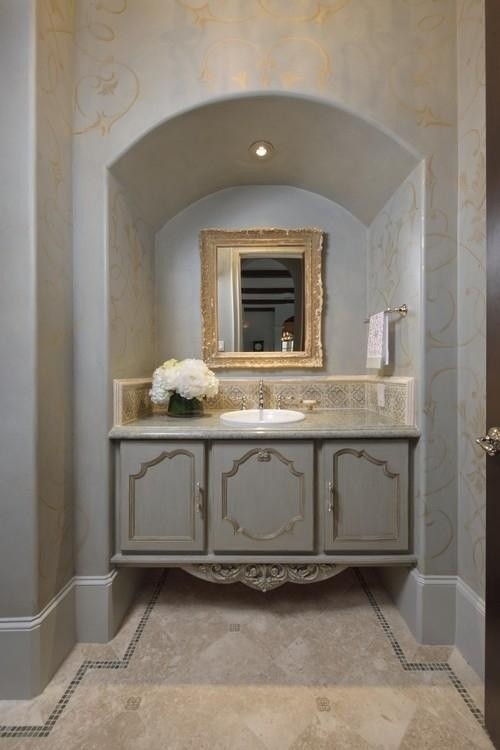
147 357 220 405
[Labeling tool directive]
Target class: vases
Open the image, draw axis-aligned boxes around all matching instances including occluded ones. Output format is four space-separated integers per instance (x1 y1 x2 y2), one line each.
166 393 204 418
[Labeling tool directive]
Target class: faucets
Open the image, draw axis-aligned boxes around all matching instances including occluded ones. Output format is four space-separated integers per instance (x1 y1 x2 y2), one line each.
258 378 264 410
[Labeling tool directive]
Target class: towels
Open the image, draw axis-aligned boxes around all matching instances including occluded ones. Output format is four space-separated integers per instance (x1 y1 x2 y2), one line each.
364 310 391 370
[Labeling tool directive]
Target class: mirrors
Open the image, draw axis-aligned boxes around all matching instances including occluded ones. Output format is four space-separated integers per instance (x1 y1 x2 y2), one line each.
199 224 327 372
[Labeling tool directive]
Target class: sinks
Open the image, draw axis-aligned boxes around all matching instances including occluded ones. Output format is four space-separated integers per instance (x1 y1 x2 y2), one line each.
220 408 307 425
